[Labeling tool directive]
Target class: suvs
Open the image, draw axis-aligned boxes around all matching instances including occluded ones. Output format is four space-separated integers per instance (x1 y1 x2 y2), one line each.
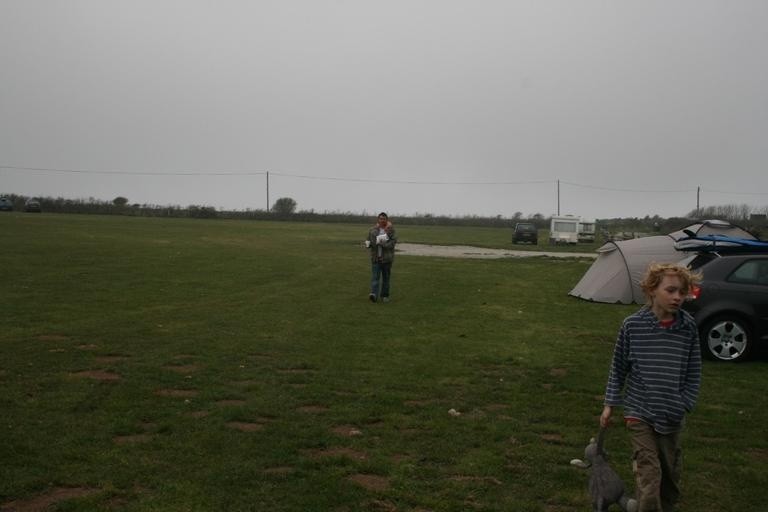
673 229 767 364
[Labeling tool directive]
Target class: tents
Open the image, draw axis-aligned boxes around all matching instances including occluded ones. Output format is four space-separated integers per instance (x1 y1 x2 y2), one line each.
568 218 755 304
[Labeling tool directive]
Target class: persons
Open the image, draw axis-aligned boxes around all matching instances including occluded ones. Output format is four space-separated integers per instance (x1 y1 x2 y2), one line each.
364 212 396 303
599 263 705 512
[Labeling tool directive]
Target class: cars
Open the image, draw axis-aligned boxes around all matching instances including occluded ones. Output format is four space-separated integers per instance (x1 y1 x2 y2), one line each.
511 223 537 245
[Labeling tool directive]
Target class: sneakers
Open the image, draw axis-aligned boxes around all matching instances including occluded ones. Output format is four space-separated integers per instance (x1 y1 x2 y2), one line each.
382 297 390 303
369 294 376 303
